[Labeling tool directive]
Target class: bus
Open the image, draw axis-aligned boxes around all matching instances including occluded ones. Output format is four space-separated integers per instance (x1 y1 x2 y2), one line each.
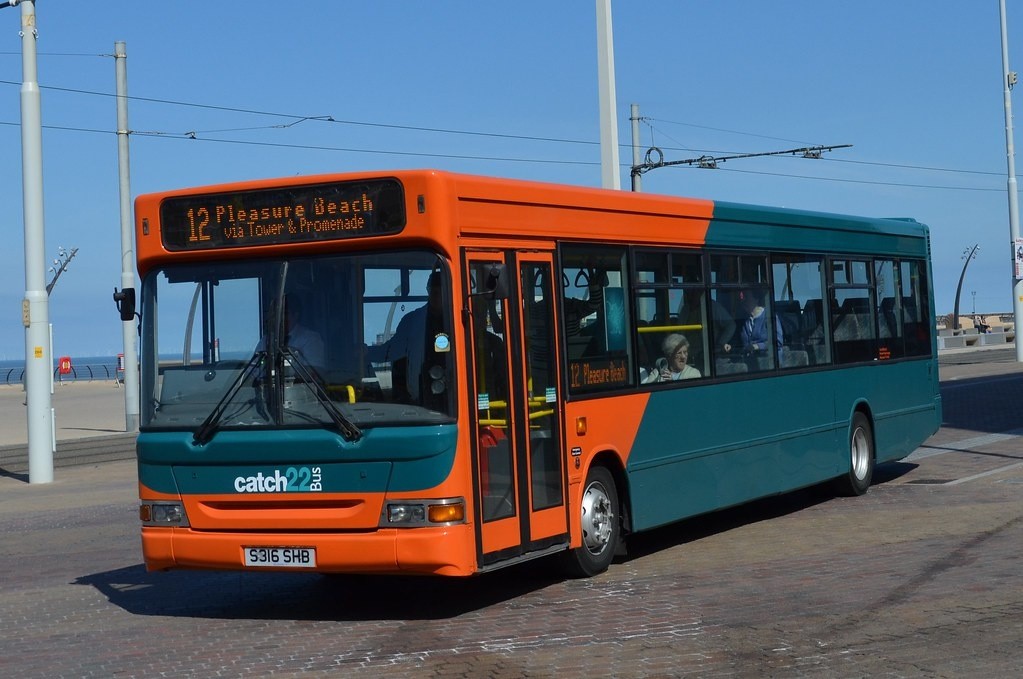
113 171 942 580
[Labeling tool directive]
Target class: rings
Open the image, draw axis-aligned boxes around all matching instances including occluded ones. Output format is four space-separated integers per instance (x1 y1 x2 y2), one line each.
669 373 672 377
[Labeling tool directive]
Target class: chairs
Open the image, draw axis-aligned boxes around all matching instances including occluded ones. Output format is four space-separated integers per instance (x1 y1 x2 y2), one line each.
633 295 918 385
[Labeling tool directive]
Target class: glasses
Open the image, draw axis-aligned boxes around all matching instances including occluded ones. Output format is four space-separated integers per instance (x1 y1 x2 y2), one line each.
668 350 688 358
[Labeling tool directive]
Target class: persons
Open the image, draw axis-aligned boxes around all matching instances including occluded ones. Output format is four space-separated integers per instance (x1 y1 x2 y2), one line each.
351 269 444 413
676 272 736 359
488 263 603 397
483 331 508 400
658 332 701 380
721 288 784 367
249 293 335 388
